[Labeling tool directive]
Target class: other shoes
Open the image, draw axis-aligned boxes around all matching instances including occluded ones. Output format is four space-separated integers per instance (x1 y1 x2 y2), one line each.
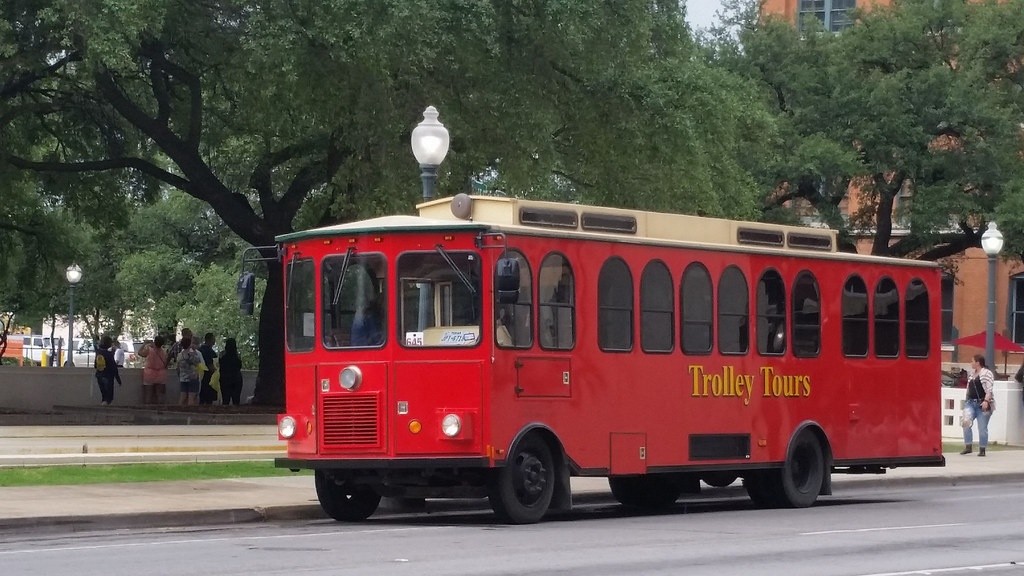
959 444 972 455
977 446 986 456
102 401 113 407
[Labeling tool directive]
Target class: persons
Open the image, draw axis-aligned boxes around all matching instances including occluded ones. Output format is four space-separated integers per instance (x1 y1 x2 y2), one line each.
94 336 122 406
111 340 125 369
959 354 997 456
136 328 244 406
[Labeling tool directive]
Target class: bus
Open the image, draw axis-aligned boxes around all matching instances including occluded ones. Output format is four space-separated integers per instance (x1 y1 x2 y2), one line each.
235 193 947 526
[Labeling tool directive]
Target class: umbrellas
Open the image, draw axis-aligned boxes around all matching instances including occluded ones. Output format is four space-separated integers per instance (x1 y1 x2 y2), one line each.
951 329 1024 352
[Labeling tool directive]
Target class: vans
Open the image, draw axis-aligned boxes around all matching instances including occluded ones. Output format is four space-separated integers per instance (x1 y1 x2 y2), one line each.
63 338 146 369
22 336 68 366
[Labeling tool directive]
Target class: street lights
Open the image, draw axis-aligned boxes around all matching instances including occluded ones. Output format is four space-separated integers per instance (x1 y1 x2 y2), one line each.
981 222 1003 373
66 261 82 367
410 105 450 332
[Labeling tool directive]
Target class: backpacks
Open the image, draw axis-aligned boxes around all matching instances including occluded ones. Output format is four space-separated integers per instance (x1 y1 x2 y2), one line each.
95 354 107 372
